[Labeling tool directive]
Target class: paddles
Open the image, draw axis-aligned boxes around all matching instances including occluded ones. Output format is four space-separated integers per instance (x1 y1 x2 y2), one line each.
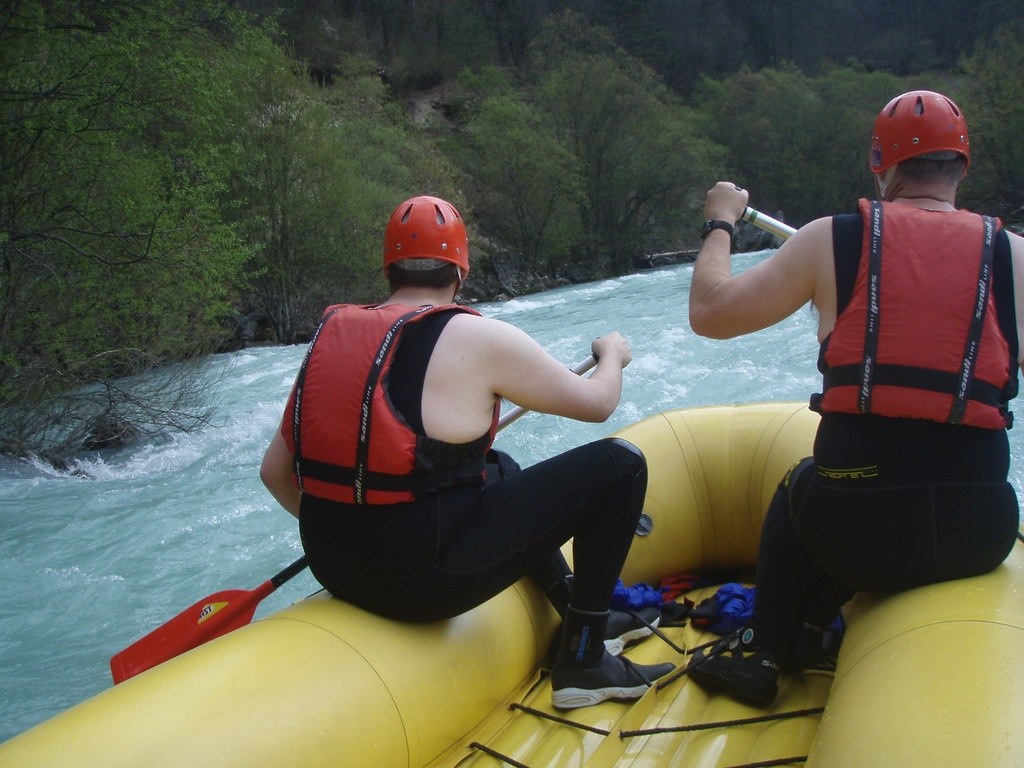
731 182 799 241
108 350 602 689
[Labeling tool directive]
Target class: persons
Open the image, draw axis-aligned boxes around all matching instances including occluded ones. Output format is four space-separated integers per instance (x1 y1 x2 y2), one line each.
259 194 676 710
688 89 1024 706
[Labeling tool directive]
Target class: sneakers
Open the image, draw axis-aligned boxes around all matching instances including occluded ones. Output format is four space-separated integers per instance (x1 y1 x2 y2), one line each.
687 622 784 710
787 612 826 668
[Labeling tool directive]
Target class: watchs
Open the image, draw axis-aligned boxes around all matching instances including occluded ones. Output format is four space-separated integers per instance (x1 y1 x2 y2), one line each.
701 219 732 238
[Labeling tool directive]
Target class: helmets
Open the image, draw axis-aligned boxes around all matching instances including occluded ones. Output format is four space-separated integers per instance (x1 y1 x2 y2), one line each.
868 91 971 182
384 195 470 286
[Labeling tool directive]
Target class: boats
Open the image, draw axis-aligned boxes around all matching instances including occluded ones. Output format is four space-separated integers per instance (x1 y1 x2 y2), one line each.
0 398 1024 768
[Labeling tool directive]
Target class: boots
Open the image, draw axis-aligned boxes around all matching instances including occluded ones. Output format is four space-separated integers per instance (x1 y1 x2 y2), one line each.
558 574 661 654
552 602 676 710
